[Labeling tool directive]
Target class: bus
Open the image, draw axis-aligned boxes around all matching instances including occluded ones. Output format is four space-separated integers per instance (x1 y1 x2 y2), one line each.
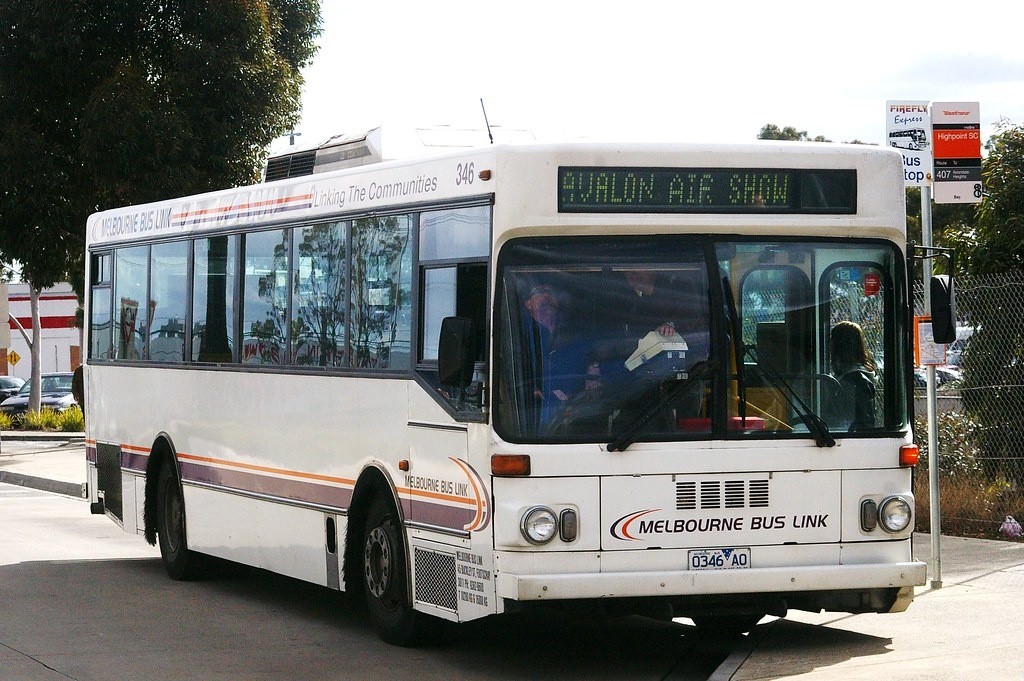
84 133 957 649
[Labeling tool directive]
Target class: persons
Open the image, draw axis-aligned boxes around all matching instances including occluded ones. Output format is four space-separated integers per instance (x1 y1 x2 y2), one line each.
71 356 85 427
827 319 879 431
511 245 714 442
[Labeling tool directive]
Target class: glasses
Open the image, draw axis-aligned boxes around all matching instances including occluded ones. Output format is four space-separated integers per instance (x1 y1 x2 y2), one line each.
528 286 558 299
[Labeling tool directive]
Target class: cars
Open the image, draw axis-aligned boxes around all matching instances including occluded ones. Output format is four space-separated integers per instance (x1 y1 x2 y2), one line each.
914 323 985 388
0 375 27 405
0 372 77 429
